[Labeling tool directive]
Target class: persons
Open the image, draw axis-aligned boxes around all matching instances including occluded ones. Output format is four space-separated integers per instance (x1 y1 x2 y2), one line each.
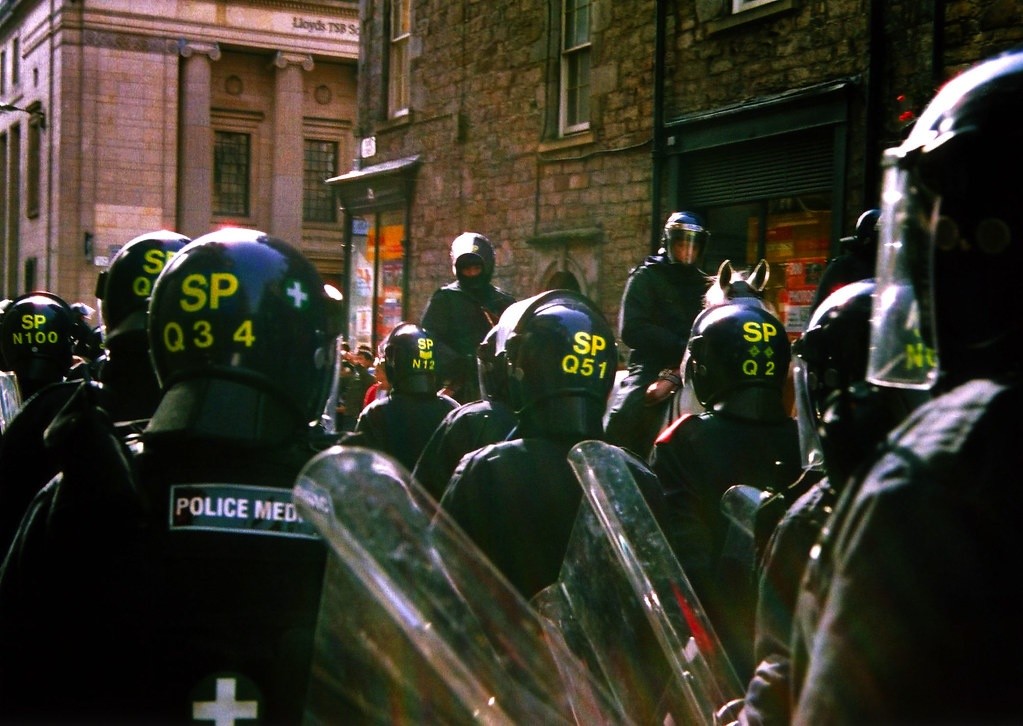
0 50 1023 726
420 231 517 405
605 210 713 446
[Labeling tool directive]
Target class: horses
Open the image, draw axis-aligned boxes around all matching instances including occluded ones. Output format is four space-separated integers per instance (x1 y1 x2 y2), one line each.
596 258 771 434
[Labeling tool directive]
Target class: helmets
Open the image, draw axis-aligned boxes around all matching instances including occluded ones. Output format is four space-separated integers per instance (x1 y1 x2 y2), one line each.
502 293 618 412
96 230 191 341
688 304 791 407
791 277 878 401
907 49 1023 257
477 324 507 401
70 304 86 318
0 291 76 365
148 228 336 425
385 323 438 384
664 211 710 258
